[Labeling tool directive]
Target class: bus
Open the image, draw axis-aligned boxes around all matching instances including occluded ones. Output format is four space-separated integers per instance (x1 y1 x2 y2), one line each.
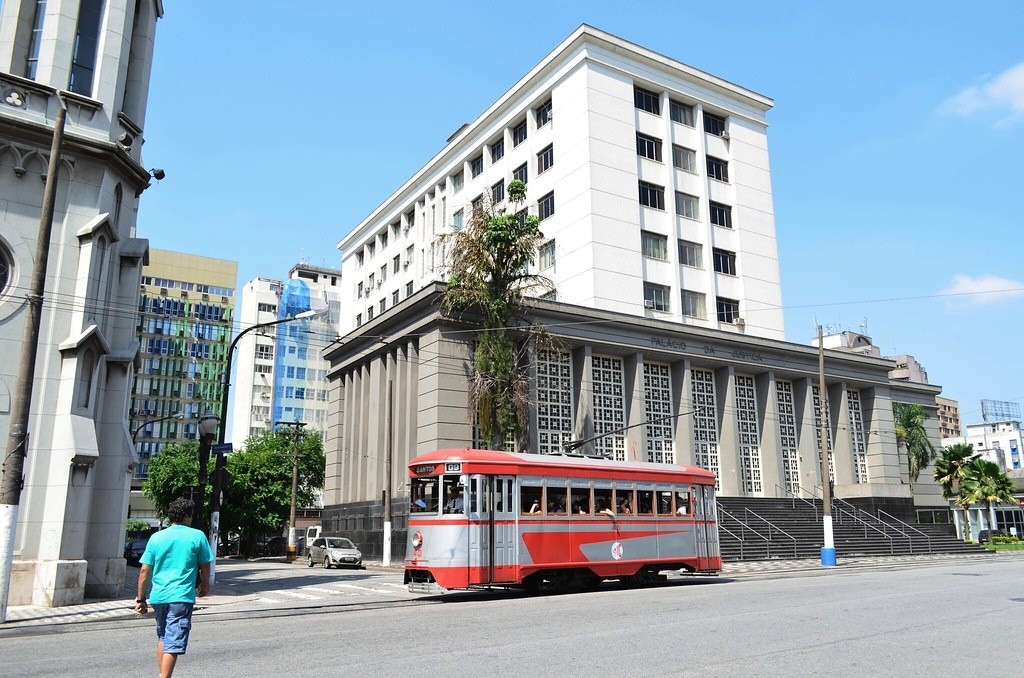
404 446 721 592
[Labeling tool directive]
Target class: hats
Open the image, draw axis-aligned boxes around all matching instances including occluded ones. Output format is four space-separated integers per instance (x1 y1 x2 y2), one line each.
450 487 460 491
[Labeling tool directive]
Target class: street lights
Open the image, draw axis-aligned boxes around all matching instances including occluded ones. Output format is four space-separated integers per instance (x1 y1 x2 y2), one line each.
191 410 220 531
208 308 328 588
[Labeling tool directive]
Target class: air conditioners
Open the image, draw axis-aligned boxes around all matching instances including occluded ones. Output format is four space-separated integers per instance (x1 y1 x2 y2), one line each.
645 299 653 307
734 318 744 325
364 287 370 292
721 130 730 140
405 225 410 230
377 279 382 284
403 260 410 266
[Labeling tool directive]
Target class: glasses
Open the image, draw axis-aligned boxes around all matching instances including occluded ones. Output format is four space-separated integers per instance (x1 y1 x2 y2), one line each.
533 502 539 504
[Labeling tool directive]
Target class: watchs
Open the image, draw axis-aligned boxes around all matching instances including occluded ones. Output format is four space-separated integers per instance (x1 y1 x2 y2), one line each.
135 597 146 603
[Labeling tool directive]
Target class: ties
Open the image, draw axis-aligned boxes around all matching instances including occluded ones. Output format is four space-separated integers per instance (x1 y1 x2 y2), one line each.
453 499 455 508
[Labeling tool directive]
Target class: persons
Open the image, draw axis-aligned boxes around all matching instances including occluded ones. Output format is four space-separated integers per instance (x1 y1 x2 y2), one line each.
448 486 464 514
497 492 686 517
135 498 215 678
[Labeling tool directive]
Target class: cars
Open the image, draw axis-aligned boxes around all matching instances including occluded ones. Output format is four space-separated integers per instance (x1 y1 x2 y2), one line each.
308 537 363 569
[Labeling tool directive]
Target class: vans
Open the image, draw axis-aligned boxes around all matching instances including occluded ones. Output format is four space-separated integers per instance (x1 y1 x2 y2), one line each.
306 526 323 549
